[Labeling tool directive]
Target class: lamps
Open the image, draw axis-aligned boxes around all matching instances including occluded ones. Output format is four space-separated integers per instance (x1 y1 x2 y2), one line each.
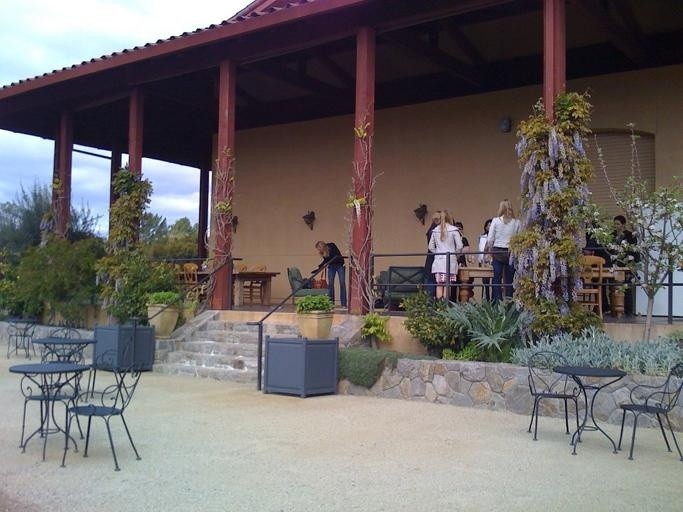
414 204 427 225
302 209 314 230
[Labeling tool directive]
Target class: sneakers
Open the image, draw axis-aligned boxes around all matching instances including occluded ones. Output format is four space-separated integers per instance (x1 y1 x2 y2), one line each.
602 308 612 314
625 314 635 319
341 305 347 309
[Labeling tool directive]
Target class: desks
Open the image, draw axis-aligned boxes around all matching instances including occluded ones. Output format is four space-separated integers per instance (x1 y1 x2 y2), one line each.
180 271 281 306
456 266 637 322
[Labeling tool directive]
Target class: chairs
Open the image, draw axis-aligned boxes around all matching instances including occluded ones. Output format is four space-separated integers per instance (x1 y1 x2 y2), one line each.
380 266 425 310
618 361 683 460
576 256 605 319
287 267 330 304
528 351 581 442
183 263 207 302
7 315 142 471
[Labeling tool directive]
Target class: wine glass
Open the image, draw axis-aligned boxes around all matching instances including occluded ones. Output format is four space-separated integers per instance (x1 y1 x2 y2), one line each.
467 254 475 267
488 256 493 265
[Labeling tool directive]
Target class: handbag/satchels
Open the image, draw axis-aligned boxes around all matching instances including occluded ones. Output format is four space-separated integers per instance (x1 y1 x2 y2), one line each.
491 248 510 264
301 278 313 289
313 279 328 289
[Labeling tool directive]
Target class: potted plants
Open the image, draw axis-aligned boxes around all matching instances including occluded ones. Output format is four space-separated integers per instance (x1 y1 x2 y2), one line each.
145 291 182 339
296 295 335 340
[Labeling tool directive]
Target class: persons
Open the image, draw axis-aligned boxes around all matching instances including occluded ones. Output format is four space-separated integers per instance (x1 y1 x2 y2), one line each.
312 240 347 309
421 194 638 320
